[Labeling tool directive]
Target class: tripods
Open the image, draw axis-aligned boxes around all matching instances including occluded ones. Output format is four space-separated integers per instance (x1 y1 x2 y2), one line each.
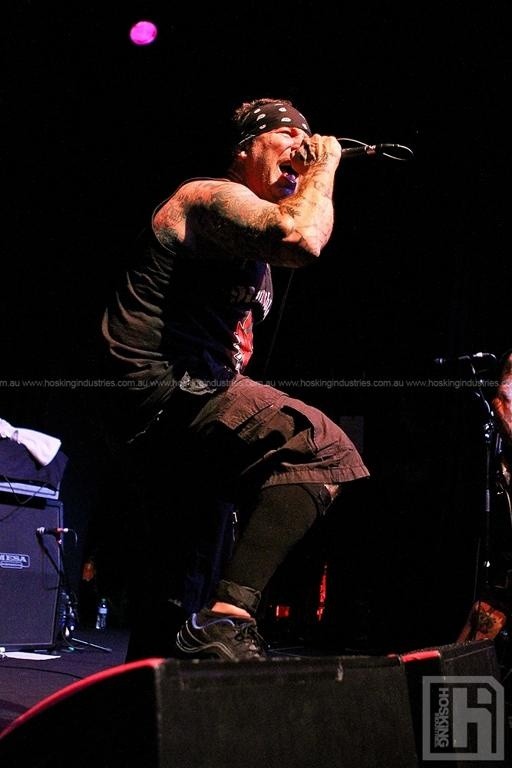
51 540 113 655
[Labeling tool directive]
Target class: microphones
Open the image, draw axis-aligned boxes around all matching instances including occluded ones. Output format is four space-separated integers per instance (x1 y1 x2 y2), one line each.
289 145 378 170
38 527 65 539
434 356 485 366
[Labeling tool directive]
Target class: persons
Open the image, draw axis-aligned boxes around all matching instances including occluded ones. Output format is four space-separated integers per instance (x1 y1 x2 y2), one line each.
72 98 376 663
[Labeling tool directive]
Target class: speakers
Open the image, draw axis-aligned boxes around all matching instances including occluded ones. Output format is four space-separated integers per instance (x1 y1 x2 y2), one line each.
0 490 65 651
399 638 502 767
0 653 417 768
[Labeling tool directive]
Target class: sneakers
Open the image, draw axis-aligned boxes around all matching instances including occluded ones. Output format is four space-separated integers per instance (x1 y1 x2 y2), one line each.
174 614 263 661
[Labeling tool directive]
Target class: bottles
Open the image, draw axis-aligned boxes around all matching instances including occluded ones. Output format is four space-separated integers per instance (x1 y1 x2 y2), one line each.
96 598 108 632
